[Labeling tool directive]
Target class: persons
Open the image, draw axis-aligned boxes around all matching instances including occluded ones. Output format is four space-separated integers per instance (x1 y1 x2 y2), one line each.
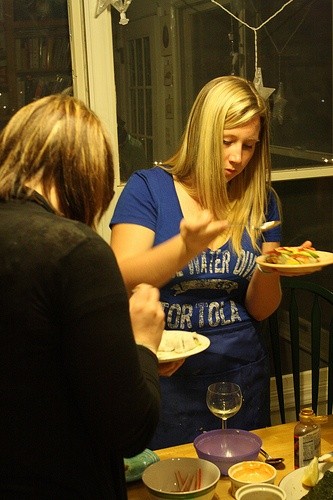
109 77 321 452
0 95 184 500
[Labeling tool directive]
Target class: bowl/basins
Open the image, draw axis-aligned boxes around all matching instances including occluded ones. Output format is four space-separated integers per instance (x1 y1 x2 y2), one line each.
227 461 277 497
234 483 286 500
194 429 262 476
142 456 220 500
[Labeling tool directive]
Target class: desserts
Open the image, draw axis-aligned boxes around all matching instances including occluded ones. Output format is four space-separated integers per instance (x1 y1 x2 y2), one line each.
158 330 200 353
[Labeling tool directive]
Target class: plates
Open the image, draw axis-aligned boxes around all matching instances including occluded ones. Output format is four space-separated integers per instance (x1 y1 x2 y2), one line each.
255 251 333 273
278 462 333 500
157 330 210 363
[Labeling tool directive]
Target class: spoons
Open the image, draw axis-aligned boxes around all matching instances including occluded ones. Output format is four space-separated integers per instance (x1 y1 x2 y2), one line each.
228 220 284 231
260 448 284 464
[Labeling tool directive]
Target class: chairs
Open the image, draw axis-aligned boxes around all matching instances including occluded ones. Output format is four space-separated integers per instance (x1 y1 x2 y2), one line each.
270 279 333 423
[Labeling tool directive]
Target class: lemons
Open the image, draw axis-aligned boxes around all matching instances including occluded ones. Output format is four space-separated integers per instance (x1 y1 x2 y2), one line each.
302 456 318 486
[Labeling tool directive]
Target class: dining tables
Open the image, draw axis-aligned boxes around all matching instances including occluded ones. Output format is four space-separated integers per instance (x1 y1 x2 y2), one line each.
127 415 333 500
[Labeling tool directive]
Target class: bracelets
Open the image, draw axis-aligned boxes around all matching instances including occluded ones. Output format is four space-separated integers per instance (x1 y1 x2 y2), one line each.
258 264 272 274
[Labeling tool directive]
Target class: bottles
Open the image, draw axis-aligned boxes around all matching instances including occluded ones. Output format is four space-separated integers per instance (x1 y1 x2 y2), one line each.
294 408 321 469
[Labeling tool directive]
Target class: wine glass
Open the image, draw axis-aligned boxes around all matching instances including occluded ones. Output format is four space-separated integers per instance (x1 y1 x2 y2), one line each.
207 382 242 429
